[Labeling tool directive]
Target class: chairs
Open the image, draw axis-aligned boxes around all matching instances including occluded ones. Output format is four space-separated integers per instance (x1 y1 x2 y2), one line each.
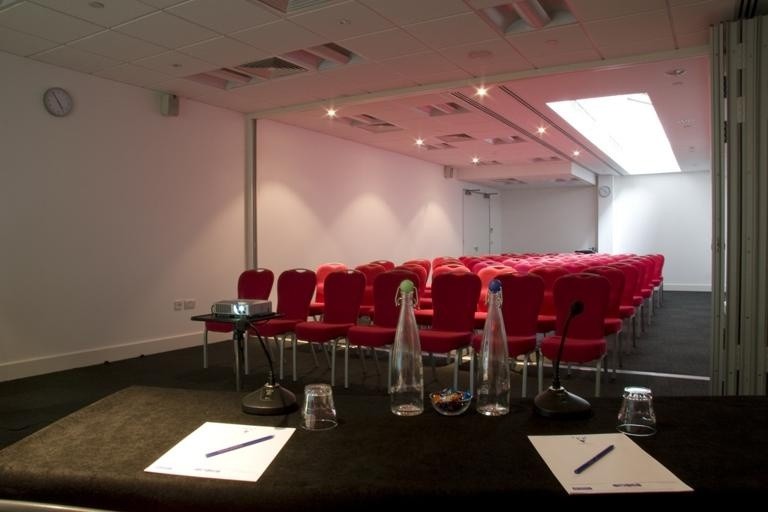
244 267 317 380
205 268 274 389
293 251 665 377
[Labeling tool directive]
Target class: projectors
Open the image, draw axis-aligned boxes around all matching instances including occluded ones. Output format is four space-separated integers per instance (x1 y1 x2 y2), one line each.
212 298 272 319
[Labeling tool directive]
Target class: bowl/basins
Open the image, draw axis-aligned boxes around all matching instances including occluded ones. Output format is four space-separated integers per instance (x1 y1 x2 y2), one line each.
428 391 473 416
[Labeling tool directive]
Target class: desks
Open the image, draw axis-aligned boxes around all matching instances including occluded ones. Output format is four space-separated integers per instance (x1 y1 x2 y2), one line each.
0 382 768 512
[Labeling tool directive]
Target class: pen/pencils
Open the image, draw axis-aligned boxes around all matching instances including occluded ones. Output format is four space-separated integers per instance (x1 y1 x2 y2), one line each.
574 444 615 475
207 436 273 457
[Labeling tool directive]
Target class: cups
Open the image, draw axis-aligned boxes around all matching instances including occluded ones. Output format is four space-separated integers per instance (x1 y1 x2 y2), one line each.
615 385 658 438
297 382 338 431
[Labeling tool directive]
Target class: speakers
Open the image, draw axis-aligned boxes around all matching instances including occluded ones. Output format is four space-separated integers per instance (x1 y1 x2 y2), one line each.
160 94 179 116
444 166 453 178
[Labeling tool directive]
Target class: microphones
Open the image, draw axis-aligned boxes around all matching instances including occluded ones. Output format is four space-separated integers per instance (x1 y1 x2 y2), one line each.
234 319 298 416
534 299 593 418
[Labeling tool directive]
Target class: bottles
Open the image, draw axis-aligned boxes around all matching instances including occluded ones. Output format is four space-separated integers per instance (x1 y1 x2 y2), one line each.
387 279 427 416
475 280 512 418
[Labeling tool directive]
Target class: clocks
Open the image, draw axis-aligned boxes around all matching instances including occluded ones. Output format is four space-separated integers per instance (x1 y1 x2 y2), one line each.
598 185 611 198
43 86 73 117
